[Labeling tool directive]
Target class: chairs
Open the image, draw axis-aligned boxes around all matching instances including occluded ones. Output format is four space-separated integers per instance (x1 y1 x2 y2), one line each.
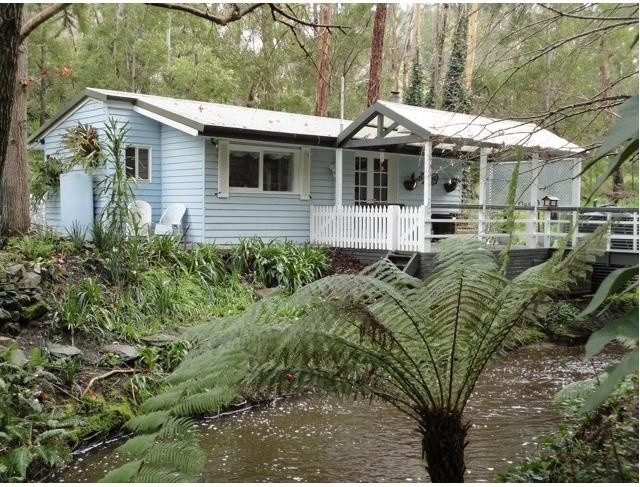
154 204 187 238
126 200 152 241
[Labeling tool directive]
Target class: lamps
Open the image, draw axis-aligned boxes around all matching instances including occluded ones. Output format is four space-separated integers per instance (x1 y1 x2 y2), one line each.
444 178 458 192
404 171 424 190
